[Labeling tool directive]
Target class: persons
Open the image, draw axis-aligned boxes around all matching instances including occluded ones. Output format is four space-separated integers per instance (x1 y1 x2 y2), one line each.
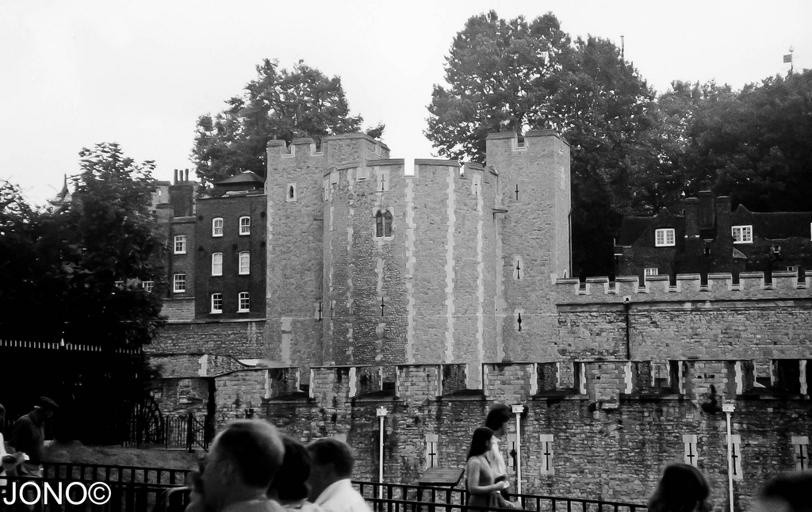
648 464 713 512
185 419 372 512
467 427 511 512
485 404 514 508
0 403 18 512
8 397 59 512
754 470 812 512
